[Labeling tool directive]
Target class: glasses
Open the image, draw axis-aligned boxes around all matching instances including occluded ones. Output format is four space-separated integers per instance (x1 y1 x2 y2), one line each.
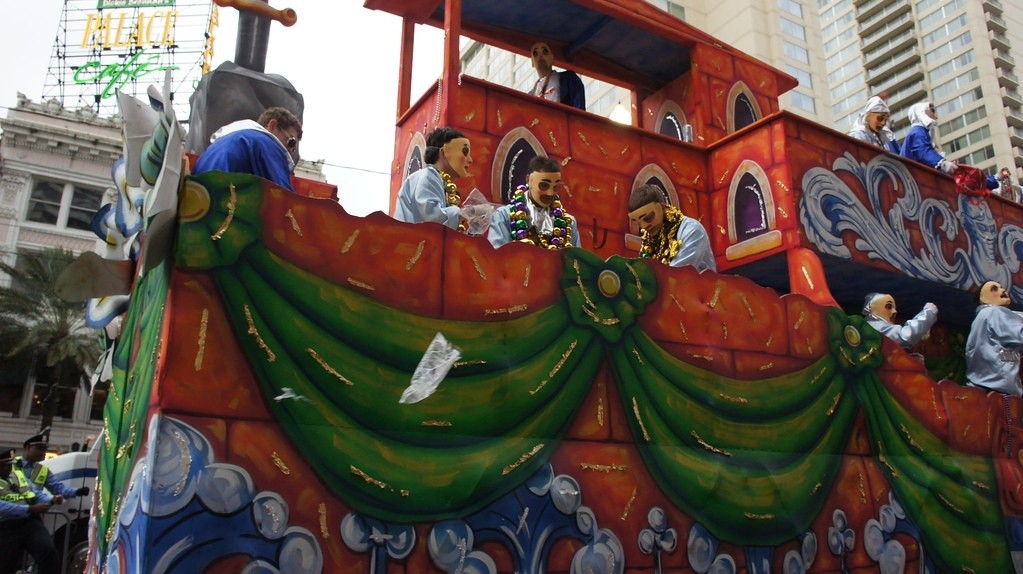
280 127 298 153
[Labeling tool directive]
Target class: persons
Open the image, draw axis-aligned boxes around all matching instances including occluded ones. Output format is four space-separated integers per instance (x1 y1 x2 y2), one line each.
628 184 716 271
393 128 495 236
528 42 586 111
992 167 1023 205
862 292 939 348
900 102 958 174
0 425 97 574
191 106 303 193
487 156 582 250
968 279 1023 398
847 96 895 152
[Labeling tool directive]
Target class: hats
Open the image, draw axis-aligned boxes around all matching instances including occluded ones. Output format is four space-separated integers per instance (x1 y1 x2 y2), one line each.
24 435 49 447
849 95 890 132
0 448 17 463
907 102 938 127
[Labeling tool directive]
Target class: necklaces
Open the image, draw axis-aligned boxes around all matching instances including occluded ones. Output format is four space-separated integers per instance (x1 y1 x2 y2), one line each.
532 73 551 98
510 183 574 249
438 168 469 233
639 204 684 265
